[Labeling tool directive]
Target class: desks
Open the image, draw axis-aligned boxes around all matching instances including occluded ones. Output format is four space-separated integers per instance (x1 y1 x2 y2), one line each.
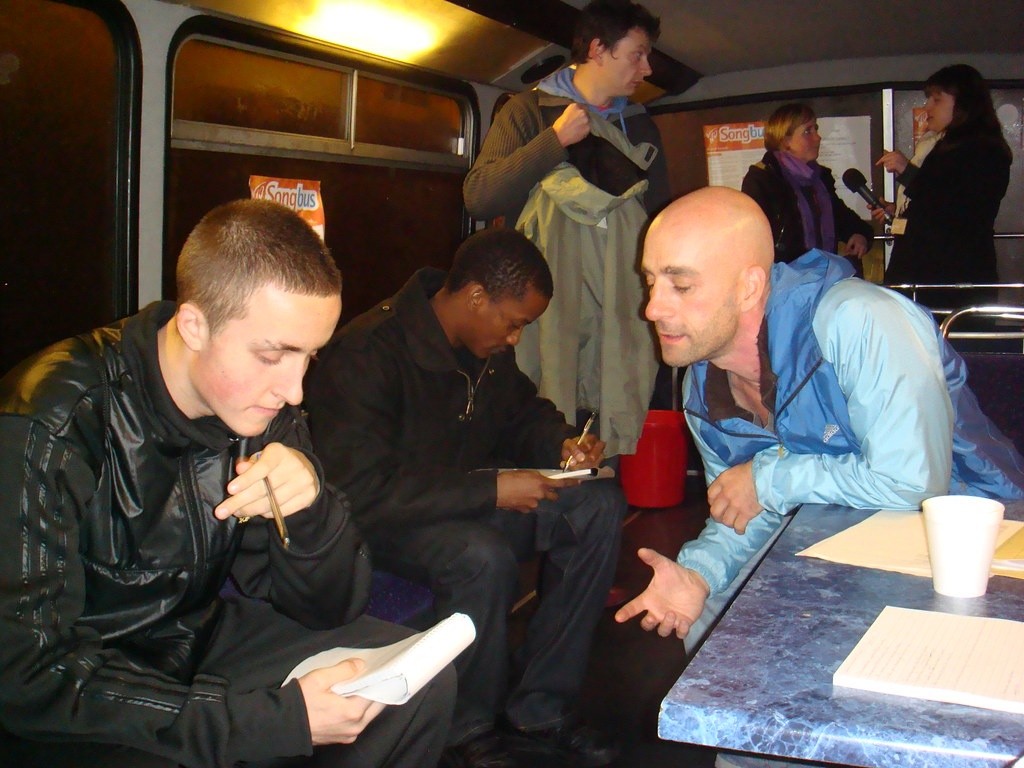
656 498 1024 768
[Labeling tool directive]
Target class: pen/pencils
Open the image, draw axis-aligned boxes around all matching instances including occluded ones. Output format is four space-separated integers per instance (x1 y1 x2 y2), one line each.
257 453 290 546
563 412 597 472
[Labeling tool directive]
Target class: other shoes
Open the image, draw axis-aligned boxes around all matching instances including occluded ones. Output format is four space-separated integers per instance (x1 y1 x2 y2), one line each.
491 711 620 768
604 586 633 608
453 728 518 768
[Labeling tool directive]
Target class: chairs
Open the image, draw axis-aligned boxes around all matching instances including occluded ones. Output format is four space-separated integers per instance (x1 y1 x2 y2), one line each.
938 305 1024 455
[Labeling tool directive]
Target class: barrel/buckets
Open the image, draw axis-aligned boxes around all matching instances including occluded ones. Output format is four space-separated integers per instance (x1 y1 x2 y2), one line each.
618 409 688 510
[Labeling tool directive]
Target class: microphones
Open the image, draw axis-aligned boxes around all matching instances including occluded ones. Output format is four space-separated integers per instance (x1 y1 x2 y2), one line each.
842 168 893 226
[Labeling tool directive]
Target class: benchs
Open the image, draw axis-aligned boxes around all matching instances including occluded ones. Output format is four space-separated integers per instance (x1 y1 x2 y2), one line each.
215 552 439 632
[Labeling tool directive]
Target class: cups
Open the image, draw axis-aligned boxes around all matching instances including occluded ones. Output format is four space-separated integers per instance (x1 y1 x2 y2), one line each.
920 494 1006 599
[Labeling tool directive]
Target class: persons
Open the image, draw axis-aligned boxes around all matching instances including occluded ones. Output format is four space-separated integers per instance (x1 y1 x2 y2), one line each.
741 103 873 264
1 199 459 768
303 229 626 768
616 187 1024 665
867 63 1013 328
463 0 674 485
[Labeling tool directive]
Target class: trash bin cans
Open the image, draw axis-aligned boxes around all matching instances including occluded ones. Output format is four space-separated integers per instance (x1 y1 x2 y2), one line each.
619 410 689 507
